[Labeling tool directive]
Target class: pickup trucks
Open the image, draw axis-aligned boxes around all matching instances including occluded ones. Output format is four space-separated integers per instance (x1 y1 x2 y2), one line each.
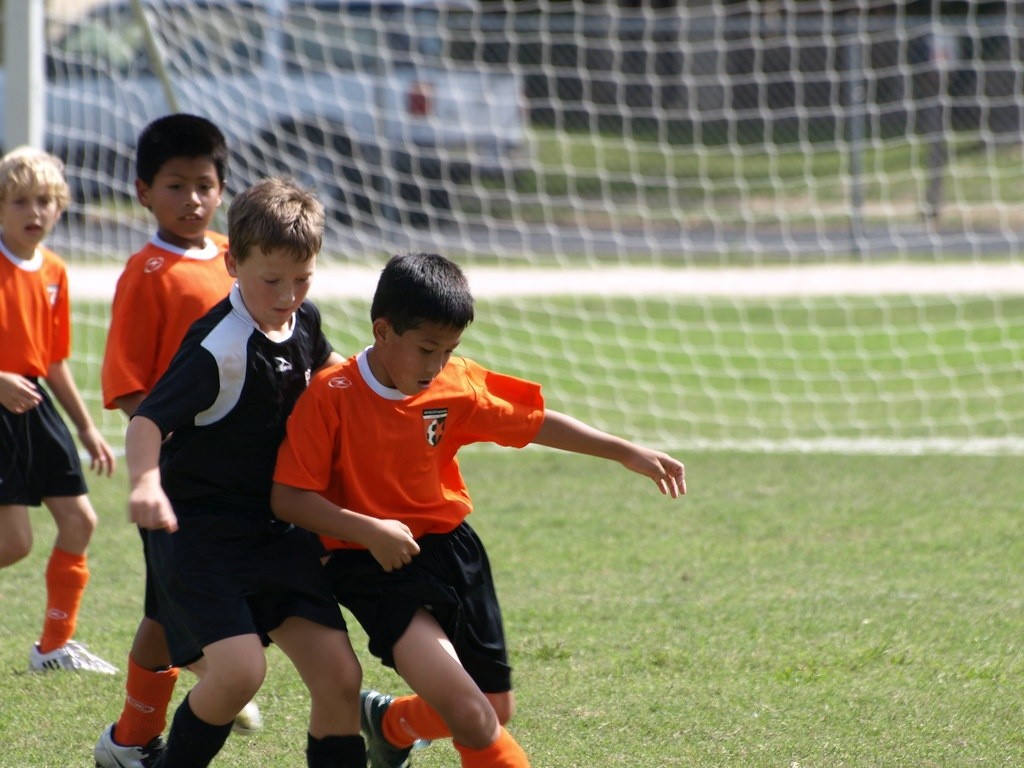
0 0 538 233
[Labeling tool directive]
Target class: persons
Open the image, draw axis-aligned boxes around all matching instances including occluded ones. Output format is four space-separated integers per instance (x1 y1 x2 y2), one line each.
0 144 117 675
96 112 263 768
271 253 687 768
127 181 367 768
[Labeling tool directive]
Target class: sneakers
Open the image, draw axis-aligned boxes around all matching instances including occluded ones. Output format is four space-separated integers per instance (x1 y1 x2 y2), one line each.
93 722 165 768
359 689 433 768
232 699 260 735
29 640 121 676
